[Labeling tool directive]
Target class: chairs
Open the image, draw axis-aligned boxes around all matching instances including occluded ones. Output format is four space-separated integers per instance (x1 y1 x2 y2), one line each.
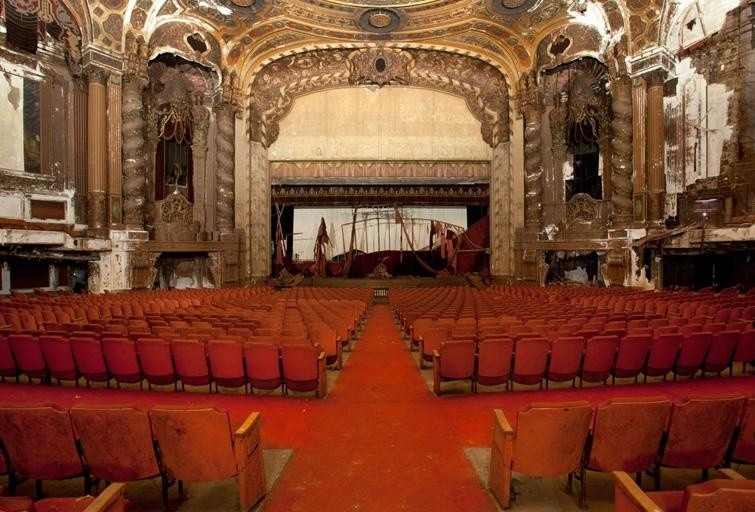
489 396 593 511
70 403 184 512
33 482 132 512
153 405 266 512
568 395 671 508
0 401 110 497
0 438 49 501
634 393 744 492
703 395 755 482
612 467 755 512
0 493 33 512
